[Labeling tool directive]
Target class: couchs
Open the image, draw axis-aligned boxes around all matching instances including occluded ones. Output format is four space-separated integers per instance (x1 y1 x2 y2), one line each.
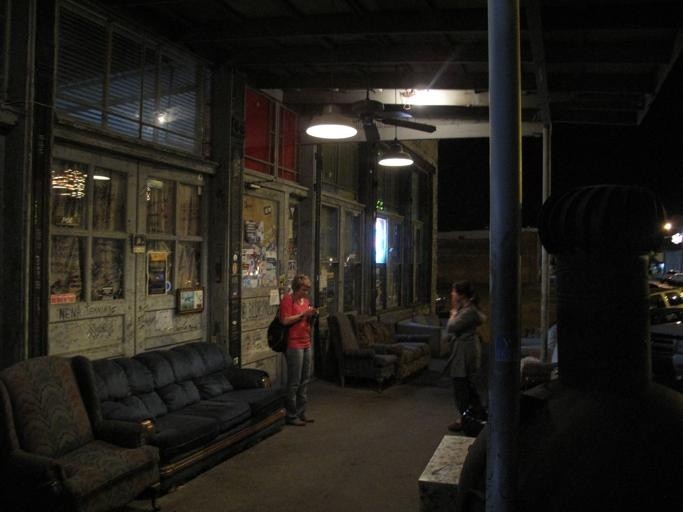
397 316 448 357
91 341 287 497
358 319 434 384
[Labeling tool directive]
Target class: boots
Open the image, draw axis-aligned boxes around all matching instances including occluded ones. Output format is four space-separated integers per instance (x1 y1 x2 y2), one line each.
286 399 305 425
296 399 313 422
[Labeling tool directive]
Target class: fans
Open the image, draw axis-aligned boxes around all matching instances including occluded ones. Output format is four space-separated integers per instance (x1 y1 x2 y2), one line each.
351 90 436 158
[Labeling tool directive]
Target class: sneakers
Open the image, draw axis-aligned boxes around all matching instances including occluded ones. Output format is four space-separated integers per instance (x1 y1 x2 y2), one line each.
448 422 461 432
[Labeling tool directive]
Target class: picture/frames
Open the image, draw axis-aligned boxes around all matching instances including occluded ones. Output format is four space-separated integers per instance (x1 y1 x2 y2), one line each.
177 287 204 314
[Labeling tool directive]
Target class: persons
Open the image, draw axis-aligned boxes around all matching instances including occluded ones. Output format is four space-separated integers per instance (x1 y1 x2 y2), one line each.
279 273 319 427
447 278 486 432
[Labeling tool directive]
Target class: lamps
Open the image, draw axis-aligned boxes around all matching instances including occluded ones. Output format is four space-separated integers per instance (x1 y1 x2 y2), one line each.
306 73 358 139
378 125 414 167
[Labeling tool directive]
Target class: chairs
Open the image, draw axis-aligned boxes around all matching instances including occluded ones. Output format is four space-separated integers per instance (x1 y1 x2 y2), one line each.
0 356 160 512
327 312 397 392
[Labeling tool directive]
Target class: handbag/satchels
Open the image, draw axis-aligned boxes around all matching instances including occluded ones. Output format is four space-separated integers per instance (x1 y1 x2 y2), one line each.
267 316 287 352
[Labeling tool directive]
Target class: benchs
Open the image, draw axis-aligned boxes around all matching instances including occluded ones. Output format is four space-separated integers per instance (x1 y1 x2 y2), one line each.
417 434 477 511
